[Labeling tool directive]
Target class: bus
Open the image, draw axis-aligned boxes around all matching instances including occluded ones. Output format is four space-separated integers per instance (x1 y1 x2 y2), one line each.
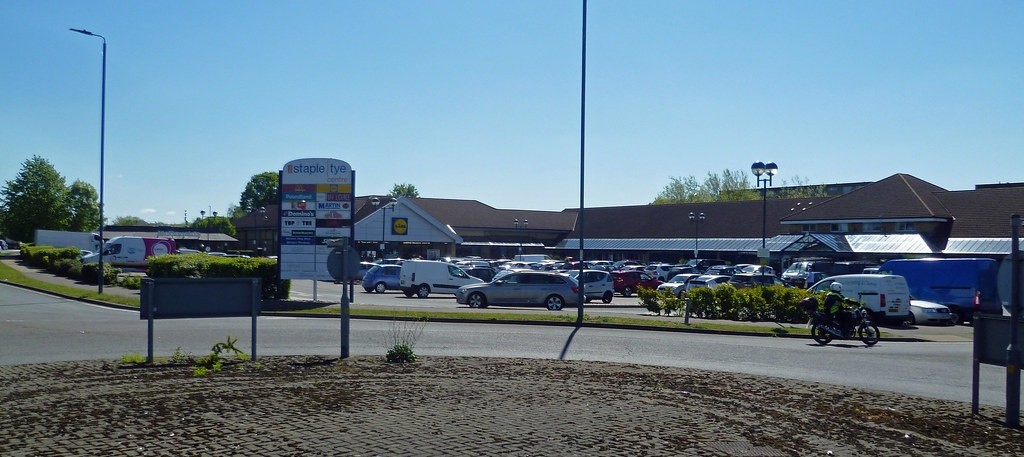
874 258 1002 326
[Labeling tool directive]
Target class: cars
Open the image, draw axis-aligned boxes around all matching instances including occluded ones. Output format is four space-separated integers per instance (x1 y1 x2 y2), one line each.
906 299 952 327
175 248 202 254
349 253 911 326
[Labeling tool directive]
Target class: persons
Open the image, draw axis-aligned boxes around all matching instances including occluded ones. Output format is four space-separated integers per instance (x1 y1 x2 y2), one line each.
823 281 861 335
562 257 574 271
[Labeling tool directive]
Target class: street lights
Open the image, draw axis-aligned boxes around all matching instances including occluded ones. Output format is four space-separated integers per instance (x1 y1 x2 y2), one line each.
199 205 218 250
68 26 110 294
206 251 225 257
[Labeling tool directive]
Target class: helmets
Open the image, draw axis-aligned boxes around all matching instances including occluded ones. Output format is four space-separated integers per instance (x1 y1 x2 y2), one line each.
830 282 842 292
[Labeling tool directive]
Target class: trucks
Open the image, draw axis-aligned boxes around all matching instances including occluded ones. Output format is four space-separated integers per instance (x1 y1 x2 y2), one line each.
33 229 105 254
79 237 175 271
